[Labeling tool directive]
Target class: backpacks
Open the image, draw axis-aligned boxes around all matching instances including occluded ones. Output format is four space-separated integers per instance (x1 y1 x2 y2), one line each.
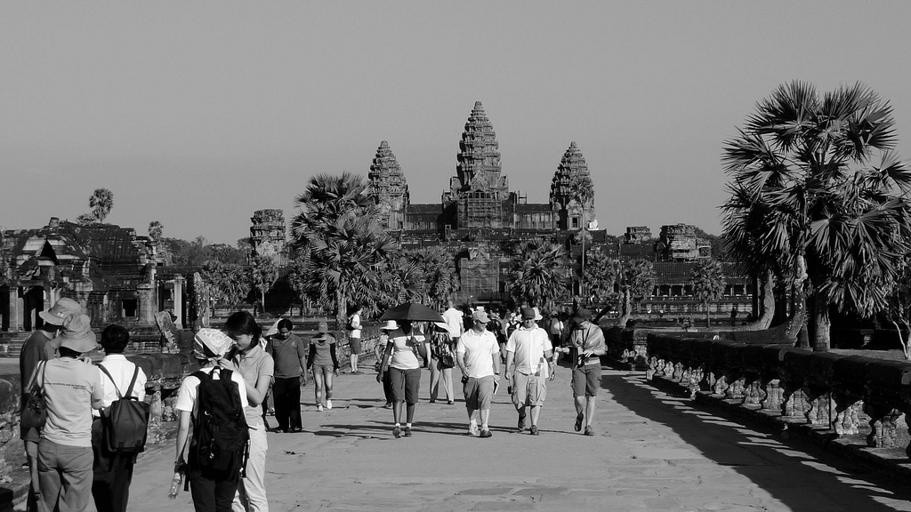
95 363 150 454
177 366 250 491
508 320 518 338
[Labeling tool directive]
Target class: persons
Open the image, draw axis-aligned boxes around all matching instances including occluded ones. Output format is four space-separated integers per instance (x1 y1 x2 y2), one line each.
307 320 340 414
377 319 428 437
555 308 609 437
16 297 86 511
266 317 307 434
175 327 248 512
218 309 276 511
506 307 555 435
347 297 597 409
91 323 149 512
456 309 503 437
27 312 105 512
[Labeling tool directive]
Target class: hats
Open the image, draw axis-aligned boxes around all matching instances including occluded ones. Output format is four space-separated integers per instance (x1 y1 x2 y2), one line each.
433 316 449 332
573 309 592 324
319 324 328 333
532 307 543 321
549 311 558 316
472 311 491 324
379 320 399 332
38 298 82 327
521 307 535 319
49 312 98 353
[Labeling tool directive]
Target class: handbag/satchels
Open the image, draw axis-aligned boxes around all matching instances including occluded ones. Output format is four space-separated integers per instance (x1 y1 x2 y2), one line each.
346 317 355 331
19 359 47 430
440 355 454 369
375 361 389 372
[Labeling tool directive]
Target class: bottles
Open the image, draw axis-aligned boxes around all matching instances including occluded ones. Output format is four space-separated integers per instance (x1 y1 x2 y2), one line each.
166 470 185 500
460 368 471 385
551 350 559 367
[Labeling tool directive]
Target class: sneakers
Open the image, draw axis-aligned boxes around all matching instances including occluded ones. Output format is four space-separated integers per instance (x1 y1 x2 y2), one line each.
585 425 594 436
575 413 584 431
468 422 479 436
385 402 393 409
326 400 332 409
480 430 492 437
393 426 401 438
316 403 323 411
530 425 539 435
518 412 526 431
405 427 411 437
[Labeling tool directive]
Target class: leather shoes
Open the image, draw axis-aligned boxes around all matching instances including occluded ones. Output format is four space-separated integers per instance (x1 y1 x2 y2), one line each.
290 427 301 432
275 426 288 433
